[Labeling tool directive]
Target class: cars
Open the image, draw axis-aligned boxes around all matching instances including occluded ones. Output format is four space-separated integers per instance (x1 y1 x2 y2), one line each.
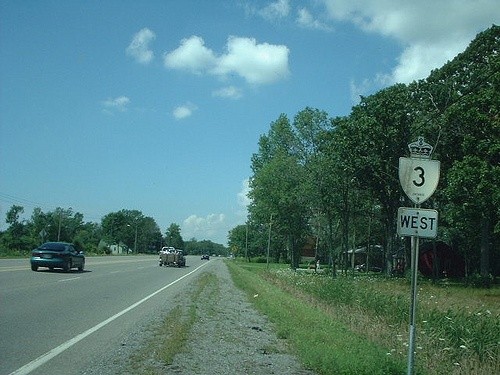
30 242 85 273
201 254 209 260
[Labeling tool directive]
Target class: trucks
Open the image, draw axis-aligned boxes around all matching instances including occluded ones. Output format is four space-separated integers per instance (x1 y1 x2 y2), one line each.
160 250 187 268
